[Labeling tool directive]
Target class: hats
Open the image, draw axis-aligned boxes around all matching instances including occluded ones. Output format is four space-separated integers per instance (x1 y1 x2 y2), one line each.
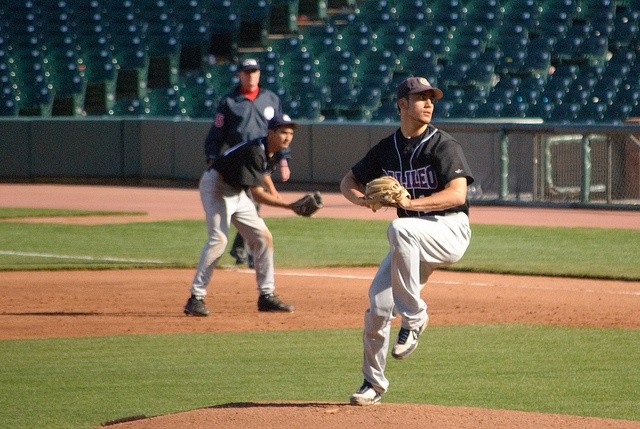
237 59 260 71
268 114 300 129
397 77 443 101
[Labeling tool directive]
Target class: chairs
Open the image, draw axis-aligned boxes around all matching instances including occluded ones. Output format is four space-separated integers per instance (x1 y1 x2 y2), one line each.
0 1 356 118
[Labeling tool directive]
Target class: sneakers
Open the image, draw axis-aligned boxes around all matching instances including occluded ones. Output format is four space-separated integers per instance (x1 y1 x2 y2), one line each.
230 248 248 261
257 294 294 312
350 379 382 405
392 317 429 360
247 254 254 268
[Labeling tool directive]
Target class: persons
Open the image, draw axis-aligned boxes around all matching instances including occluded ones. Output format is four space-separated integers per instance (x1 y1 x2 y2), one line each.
341 76 473 405
183 115 297 318
207 58 292 266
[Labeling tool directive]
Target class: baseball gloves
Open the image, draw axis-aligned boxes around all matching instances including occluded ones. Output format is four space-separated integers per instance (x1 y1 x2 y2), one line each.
291 190 323 218
363 176 411 213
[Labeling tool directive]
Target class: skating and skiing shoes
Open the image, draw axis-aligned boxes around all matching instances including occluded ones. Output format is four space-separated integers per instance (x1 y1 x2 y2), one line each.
184 297 211 316
